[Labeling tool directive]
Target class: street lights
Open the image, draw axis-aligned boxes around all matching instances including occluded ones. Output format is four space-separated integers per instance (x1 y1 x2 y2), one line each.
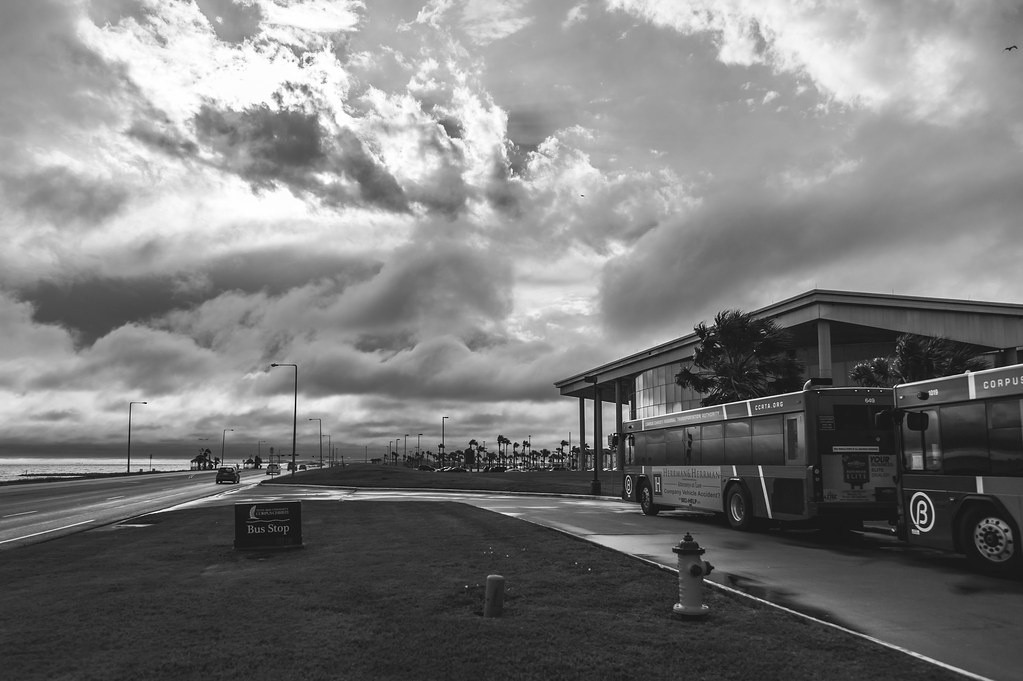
322 435 331 468
309 418 322 470
128 402 147 472
259 440 265 459
529 434 531 468
222 429 235 465
271 364 297 461
386 417 448 467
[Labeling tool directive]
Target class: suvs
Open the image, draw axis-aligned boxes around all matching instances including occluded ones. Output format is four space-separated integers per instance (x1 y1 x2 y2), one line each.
419 465 435 472
287 462 297 471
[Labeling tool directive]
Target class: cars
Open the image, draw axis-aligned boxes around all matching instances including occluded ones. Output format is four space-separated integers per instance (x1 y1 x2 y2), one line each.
299 465 307 470
483 466 617 472
265 464 281 474
437 467 466 472
214 467 241 484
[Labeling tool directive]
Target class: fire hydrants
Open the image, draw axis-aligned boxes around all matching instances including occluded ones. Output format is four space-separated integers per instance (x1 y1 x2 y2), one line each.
671 533 714 621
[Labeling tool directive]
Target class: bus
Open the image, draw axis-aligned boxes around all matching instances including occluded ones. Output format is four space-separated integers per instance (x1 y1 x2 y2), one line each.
612 380 896 532
874 363 1023 577
